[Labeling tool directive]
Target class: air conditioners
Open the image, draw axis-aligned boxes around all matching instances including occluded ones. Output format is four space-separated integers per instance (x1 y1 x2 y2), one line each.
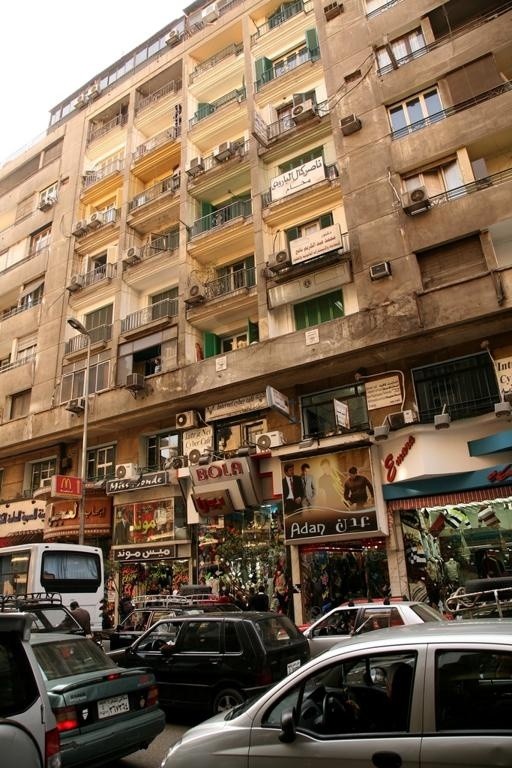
182 284 205 306
185 443 212 463
287 98 431 216
175 410 196 428
66 398 86 414
256 431 285 451
71 2 238 176
125 373 144 392
38 193 102 239
268 248 292 273
66 274 82 293
388 410 415 429
115 463 138 480
125 246 142 265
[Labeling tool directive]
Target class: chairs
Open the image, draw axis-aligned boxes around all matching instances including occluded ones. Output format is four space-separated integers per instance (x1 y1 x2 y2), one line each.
360 660 409 727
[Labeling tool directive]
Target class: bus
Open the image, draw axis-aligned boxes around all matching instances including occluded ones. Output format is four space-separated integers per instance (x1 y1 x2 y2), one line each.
0 543 105 639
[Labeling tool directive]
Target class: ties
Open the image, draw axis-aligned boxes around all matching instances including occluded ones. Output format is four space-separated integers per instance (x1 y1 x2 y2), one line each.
289 478 294 493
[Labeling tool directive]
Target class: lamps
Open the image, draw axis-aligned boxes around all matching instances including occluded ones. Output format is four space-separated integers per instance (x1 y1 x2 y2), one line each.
432 389 511 431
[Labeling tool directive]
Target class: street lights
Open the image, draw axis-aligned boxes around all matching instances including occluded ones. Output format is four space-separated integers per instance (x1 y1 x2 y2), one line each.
65 314 92 544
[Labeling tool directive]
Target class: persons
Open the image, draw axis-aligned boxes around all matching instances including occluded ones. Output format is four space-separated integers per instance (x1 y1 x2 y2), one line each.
347 660 413 733
63 600 93 635
281 462 304 516
298 464 316 511
342 466 373 508
155 357 162 373
113 507 132 544
155 576 291 616
316 458 344 508
159 643 174 655
153 501 168 532
